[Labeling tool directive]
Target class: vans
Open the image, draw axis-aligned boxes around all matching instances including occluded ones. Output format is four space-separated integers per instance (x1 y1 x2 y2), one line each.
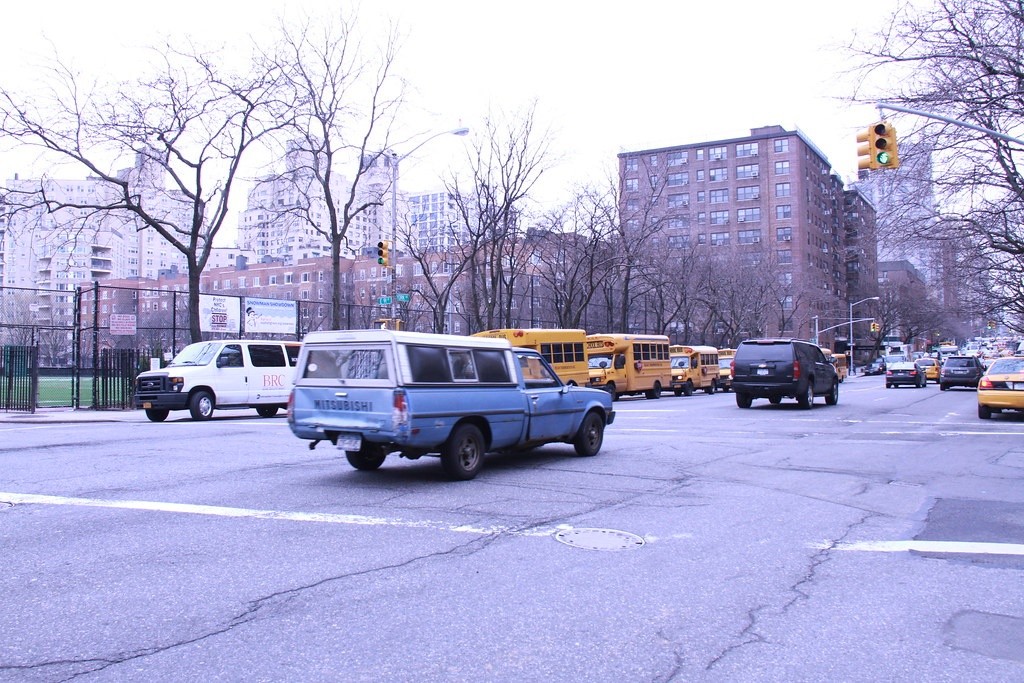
132 340 303 424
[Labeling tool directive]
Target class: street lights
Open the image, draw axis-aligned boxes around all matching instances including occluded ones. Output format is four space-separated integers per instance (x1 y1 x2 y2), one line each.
390 127 470 330
849 297 880 376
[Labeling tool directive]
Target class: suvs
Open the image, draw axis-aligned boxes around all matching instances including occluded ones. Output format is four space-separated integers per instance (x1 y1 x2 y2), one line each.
728 337 838 409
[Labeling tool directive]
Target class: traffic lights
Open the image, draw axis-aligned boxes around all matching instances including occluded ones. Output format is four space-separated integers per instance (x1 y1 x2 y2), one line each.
992 320 996 329
875 324 880 332
870 122 900 170
870 322 875 333
377 241 389 267
373 319 389 330
856 128 875 172
395 319 406 331
986 321 992 330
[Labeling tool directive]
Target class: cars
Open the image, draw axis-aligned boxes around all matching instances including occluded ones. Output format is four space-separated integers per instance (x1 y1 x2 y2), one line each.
863 335 1024 391
976 354 1024 419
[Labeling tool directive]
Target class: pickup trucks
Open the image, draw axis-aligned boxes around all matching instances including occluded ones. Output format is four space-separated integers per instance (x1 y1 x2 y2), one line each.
288 326 616 482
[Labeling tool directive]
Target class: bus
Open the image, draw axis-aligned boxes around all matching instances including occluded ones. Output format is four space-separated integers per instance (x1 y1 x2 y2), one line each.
717 347 739 392
466 328 590 387
586 332 672 403
670 344 721 396
821 348 848 384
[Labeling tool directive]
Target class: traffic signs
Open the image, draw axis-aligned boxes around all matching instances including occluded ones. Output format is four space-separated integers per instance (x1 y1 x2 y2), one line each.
396 294 410 302
376 296 392 305
